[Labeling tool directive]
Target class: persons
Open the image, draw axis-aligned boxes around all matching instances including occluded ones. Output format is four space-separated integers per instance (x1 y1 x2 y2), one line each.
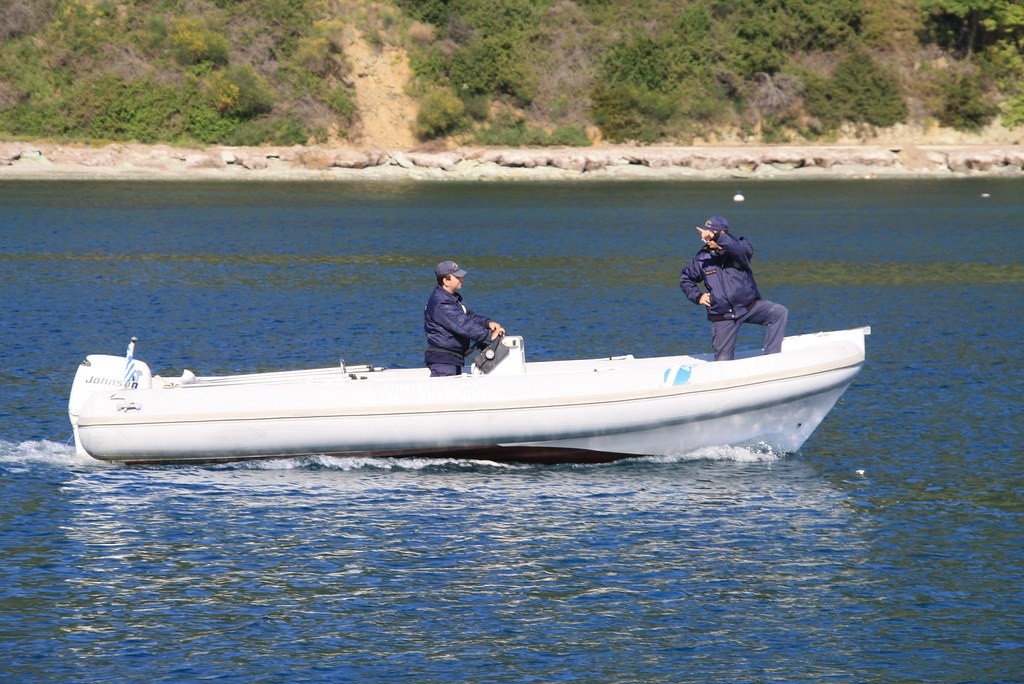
424 261 505 378
679 215 788 362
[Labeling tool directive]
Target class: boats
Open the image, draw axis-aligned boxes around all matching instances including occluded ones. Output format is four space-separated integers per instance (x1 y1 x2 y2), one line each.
68 324 877 466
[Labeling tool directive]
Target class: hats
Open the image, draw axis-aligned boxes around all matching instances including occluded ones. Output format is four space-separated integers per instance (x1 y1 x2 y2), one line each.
698 216 728 234
435 261 467 277
